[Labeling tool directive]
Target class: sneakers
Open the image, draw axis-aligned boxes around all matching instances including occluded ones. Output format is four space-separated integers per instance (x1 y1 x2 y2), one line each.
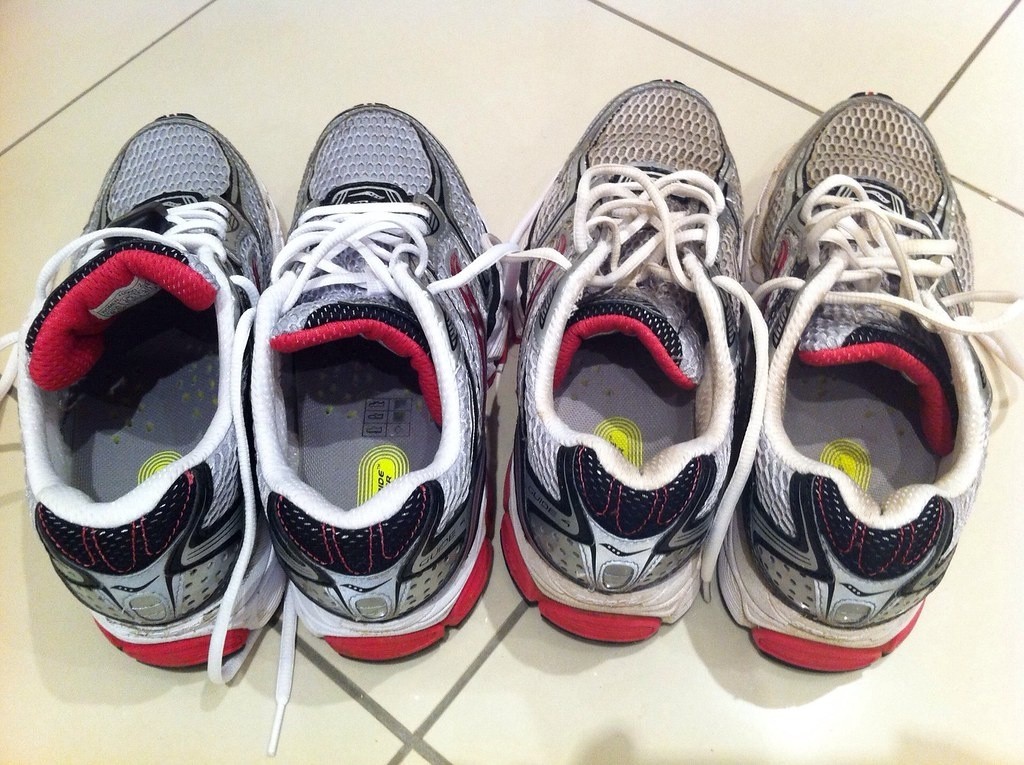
1 114 289 683
500 79 769 646
717 95 1024 674
249 103 507 757
